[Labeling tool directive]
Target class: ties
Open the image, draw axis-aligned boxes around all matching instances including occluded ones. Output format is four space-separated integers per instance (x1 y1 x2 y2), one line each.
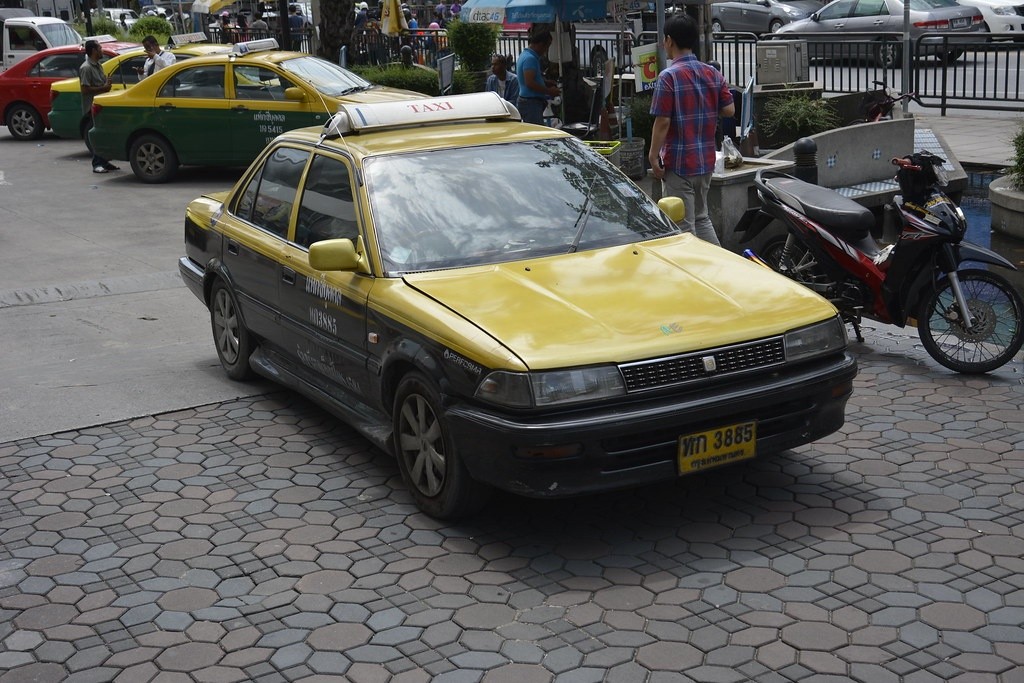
148 52 163 75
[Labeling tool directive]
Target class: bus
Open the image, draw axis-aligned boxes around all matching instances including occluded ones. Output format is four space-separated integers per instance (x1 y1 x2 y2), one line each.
495 8 682 78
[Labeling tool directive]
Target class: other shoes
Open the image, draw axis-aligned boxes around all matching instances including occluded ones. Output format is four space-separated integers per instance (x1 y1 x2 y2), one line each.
93 165 109 173
103 163 116 170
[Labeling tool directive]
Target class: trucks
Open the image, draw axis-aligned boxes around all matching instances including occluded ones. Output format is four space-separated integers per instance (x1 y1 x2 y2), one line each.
0 7 82 74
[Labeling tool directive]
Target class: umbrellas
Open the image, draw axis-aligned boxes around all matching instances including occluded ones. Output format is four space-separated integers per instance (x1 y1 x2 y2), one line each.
459 0 607 120
190 0 235 14
380 0 409 38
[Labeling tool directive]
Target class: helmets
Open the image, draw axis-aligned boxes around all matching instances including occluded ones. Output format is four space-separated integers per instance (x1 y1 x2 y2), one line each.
402 8 411 18
358 1 368 10
407 19 418 28
401 3 409 9
429 22 439 33
433 10 442 19
367 8 379 18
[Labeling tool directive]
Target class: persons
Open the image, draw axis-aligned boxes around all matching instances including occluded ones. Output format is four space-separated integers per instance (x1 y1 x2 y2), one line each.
356 0 462 68
648 13 737 247
79 40 121 173
516 28 559 124
117 13 128 32
138 36 177 82
485 54 520 105
288 5 304 50
209 11 268 41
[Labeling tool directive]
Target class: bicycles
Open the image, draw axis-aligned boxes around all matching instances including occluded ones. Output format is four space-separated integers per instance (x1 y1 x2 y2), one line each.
847 81 922 129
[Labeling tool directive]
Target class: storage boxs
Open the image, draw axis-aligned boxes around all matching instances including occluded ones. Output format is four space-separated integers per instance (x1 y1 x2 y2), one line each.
582 141 621 169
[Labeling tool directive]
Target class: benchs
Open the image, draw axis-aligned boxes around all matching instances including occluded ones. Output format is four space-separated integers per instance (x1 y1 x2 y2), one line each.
834 123 969 239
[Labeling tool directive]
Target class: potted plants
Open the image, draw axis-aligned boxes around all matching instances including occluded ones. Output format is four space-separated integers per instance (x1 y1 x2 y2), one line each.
445 18 504 81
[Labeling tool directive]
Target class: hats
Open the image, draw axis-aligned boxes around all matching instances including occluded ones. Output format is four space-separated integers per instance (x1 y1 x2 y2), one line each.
219 10 230 17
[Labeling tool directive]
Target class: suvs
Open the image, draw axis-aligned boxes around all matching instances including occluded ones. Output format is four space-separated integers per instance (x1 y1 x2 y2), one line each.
90 8 141 29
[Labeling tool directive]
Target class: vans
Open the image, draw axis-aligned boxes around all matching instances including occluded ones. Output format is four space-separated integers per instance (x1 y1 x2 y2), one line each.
140 3 180 20
290 2 313 25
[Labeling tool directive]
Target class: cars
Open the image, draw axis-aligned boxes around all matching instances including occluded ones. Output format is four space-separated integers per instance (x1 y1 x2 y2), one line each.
88 38 436 183
957 1 1024 49
710 0 829 39
772 0 986 70
0 33 146 141
166 13 189 27
179 90 860 522
48 30 345 154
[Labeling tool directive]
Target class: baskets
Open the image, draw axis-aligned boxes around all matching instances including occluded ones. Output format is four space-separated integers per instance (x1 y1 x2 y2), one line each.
583 140 622 169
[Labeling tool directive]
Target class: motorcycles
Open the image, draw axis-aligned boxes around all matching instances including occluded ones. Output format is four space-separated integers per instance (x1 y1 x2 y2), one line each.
732 148 1024 373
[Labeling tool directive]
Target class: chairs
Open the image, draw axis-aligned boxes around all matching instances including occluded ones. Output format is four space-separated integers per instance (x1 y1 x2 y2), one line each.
167 78 190 96
258 67 285 100
218 72 251 99
562 86 601 140
198 72 223 98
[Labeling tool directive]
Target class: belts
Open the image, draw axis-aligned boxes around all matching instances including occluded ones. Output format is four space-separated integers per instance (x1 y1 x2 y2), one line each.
519 96 545 99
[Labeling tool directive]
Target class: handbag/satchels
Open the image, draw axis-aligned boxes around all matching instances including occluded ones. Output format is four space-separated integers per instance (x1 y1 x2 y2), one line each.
720 135 743 169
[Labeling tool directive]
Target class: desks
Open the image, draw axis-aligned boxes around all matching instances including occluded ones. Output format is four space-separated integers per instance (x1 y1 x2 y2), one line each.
595 73 635 82
